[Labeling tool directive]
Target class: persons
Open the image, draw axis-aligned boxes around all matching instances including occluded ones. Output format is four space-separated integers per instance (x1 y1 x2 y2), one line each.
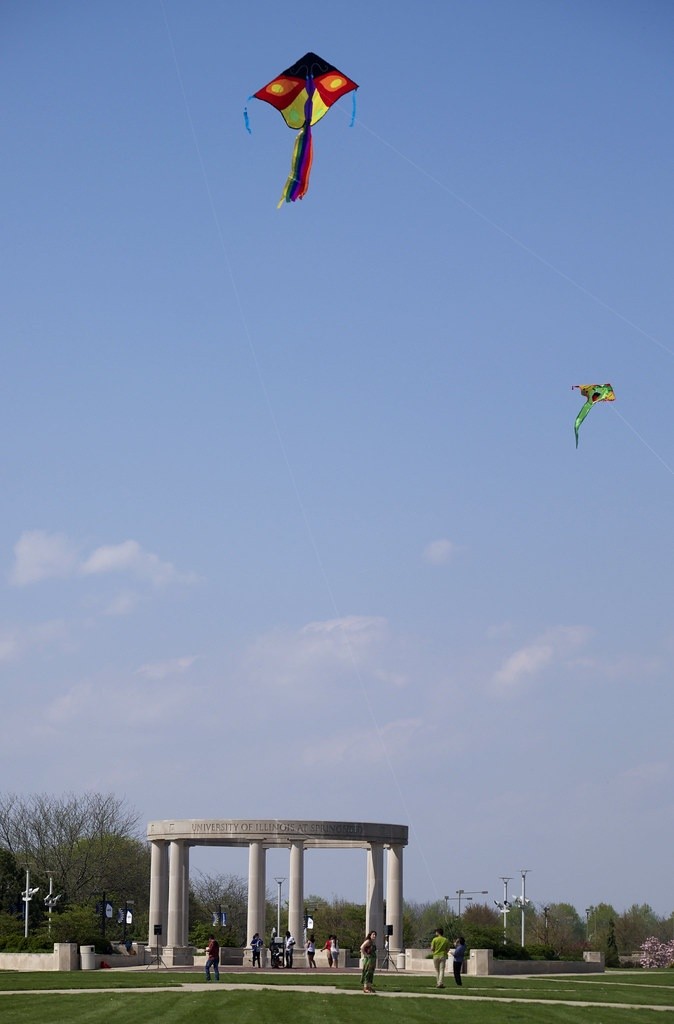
449 937 465 985
430 929 450 988
205 935 220 981
320 935 333 967
269 932 279 968
330 936 339 967
361 931 377 993
284 931 296 968
250 933 263 968
307 936 317 968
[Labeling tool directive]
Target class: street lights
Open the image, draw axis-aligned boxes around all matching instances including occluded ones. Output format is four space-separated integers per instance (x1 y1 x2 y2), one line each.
456 890 488 916
444 895 472 909
585 908 591 940
20 862 33 938
513 869 532 947
544 907 552 944
494 876 515 944
274 876 288 937
45 871 55 932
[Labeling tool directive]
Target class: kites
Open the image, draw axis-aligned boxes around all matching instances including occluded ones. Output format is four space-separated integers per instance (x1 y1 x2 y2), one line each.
568 384 616 447
245 52 359 208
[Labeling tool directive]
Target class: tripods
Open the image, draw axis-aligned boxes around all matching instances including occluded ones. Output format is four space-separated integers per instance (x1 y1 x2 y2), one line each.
146 935 167 969
380 935 398 972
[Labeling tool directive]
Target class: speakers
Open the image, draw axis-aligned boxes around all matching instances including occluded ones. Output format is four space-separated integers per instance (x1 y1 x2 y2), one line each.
154 924 162 935
384 925 393 935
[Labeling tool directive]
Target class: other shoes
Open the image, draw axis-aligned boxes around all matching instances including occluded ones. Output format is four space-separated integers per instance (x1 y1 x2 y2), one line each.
436 984 446 988
363 986 375 993
